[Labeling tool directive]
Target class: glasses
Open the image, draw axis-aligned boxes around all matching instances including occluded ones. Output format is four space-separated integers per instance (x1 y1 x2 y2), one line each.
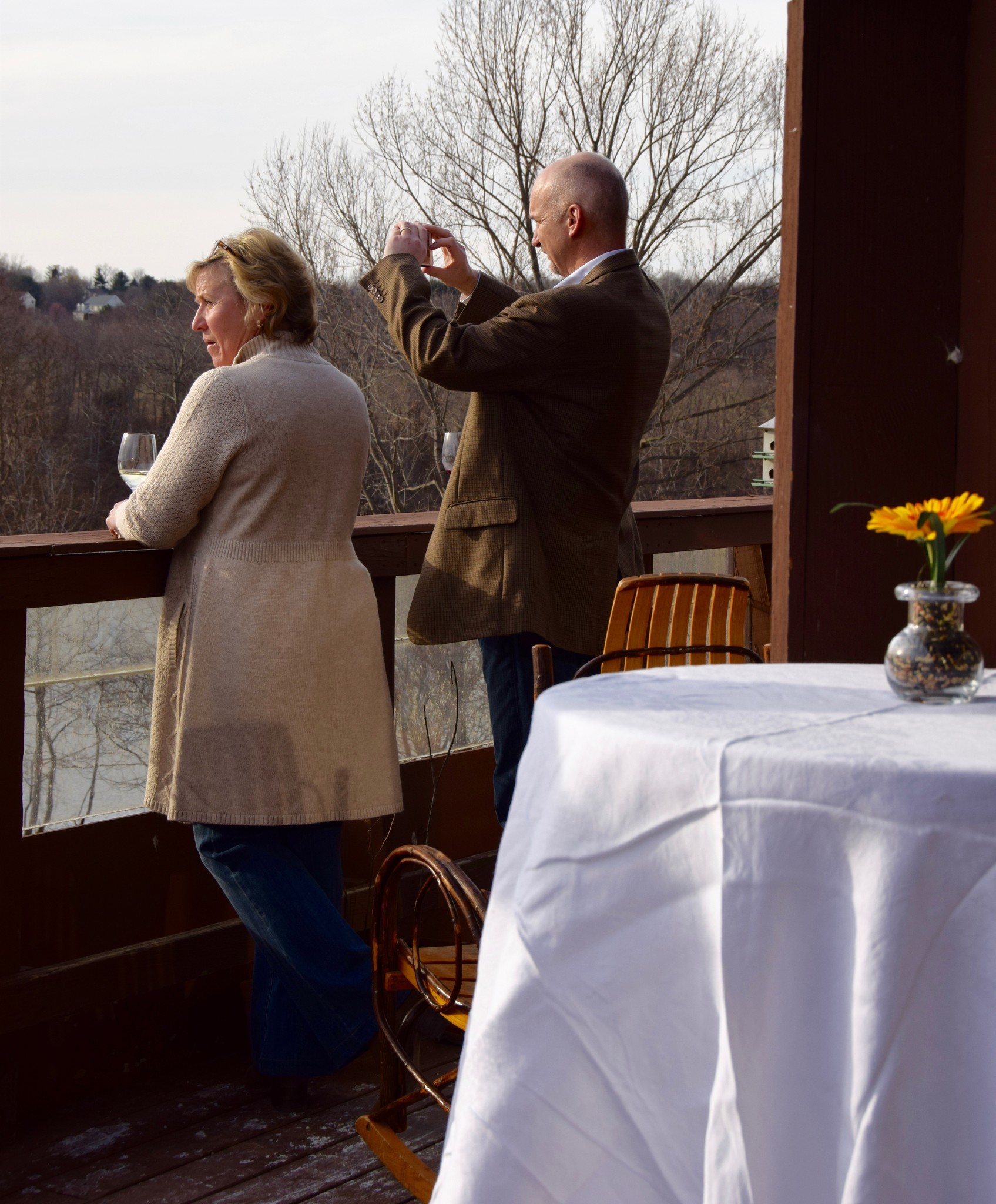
207 239 251 265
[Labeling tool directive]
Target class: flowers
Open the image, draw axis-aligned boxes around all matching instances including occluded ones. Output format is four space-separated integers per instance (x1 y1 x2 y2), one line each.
828 488 996 587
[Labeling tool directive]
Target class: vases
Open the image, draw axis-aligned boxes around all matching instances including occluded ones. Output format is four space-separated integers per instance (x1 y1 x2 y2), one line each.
884 580 984 705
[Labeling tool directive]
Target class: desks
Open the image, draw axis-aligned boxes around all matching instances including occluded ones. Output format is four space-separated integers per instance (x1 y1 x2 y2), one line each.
431 662 996 1204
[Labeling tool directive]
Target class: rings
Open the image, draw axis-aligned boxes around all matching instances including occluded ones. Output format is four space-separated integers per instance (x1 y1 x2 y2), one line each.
400 227 411 233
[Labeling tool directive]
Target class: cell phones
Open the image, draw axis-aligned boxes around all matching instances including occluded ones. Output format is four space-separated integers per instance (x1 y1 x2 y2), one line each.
420 232 434 267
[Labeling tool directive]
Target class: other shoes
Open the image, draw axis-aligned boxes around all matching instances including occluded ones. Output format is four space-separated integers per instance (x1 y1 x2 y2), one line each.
243 1060 305 1113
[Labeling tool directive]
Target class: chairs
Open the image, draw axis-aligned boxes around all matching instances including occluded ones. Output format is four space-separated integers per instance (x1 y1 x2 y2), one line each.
354 570 770 1204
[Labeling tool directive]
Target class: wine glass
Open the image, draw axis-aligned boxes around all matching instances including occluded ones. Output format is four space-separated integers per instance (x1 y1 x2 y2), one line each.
117 433 157 492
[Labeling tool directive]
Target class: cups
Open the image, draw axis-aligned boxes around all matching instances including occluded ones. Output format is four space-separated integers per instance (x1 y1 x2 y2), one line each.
442 432 462 475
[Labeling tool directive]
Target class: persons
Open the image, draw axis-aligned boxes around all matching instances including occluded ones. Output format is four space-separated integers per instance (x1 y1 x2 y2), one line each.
359 153 671 829
106 227 404 1113
